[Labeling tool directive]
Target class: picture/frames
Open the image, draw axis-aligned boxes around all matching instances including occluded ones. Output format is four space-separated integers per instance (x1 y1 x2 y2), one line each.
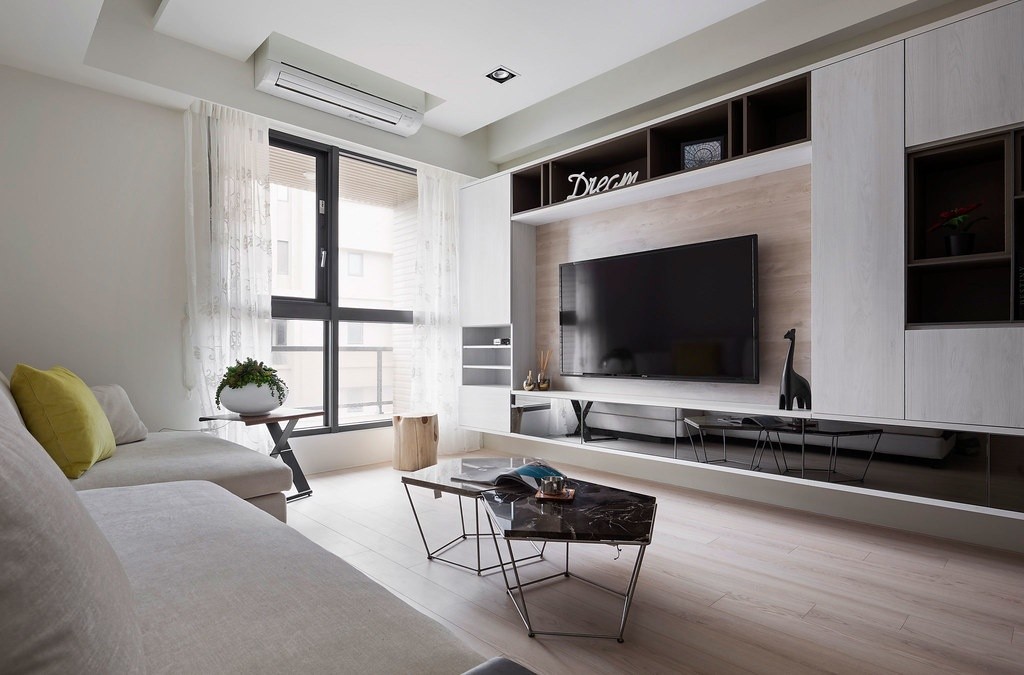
680 135 725 171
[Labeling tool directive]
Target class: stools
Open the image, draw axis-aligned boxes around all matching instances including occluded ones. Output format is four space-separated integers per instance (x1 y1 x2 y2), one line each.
391 413 439 472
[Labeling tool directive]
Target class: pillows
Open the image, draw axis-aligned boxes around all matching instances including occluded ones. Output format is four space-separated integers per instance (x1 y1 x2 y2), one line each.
10 363 116 479
88 384 148 446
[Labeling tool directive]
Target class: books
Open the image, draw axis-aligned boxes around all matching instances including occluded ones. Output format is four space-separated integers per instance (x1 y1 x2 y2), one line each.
450 458 567 492
717 413 789 430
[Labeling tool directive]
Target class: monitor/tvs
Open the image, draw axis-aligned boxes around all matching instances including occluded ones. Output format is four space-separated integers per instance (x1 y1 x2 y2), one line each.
560 233 760 385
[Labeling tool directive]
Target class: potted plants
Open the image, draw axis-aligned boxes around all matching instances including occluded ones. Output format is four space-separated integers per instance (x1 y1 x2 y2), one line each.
214 357 290 417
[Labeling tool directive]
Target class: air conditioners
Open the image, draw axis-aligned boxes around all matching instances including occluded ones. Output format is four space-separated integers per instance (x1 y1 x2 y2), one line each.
254 34 426 137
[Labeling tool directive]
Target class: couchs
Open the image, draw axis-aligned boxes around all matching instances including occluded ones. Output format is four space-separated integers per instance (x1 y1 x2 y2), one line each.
0 363 537 675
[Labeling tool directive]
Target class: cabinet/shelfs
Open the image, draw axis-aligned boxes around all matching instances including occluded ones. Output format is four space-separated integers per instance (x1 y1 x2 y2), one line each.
455 0 1024 522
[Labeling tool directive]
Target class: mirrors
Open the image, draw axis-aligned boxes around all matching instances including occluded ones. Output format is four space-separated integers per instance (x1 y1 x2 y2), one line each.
510 393 1024 515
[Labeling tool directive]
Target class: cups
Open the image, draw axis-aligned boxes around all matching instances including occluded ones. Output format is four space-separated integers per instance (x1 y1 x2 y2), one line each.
540 476 564 495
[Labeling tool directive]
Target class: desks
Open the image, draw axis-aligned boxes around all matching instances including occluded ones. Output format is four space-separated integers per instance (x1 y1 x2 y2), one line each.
200 410 325 503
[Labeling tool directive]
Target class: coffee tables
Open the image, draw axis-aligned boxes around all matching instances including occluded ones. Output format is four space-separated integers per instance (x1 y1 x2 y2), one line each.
683 415 782 471
480 477 658 644
400 456 566 575
766 420 883 485
